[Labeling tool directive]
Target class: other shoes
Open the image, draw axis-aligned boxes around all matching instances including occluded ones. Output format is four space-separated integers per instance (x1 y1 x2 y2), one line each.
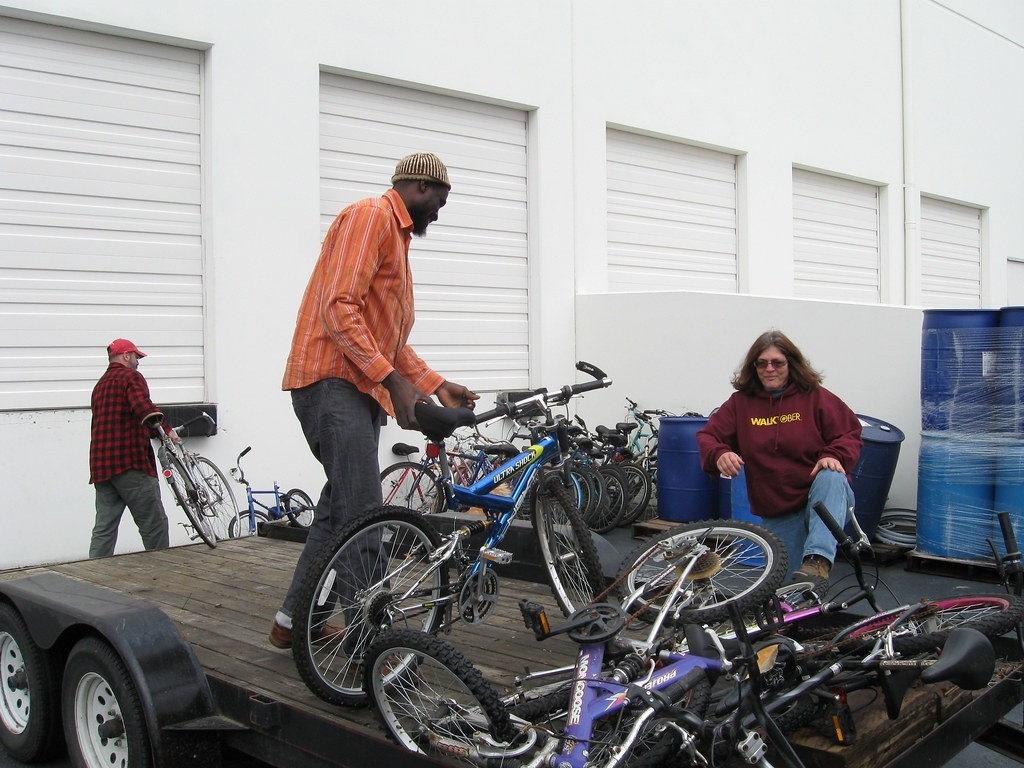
793 556 831 580
347 654 422 685
268 619 346 649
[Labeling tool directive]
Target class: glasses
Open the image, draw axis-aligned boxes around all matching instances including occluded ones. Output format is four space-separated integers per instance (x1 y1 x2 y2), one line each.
753 359 787 367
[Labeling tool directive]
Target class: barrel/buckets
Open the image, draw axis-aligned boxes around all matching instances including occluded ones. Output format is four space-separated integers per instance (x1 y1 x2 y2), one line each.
729 468 807 569
656 417 728 523
917 307 1024 565
845 414 906 544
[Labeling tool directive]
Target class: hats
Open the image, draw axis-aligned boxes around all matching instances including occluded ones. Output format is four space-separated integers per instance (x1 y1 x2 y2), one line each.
108 339 147 359
392 153 451 191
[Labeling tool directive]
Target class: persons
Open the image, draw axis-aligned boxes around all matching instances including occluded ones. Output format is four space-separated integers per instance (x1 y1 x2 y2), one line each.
88 338 181 559
268 152 482 649
695 331 866 608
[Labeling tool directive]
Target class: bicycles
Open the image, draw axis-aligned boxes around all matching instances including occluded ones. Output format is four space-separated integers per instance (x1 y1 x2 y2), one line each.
362 500 1023 768
228 446 317 538
290 361 613 708
380 397 703 534
140 411 241 549
